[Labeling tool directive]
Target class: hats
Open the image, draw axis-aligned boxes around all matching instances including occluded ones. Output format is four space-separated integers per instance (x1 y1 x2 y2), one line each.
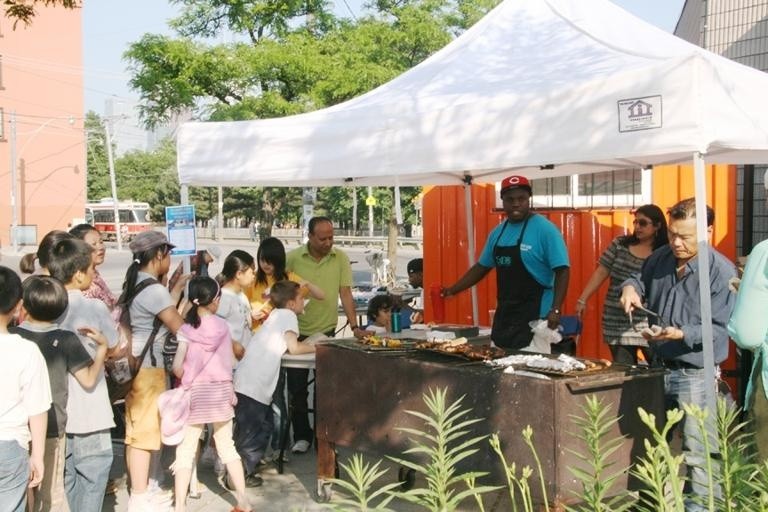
407 259 423 273
206 246 221 261
501 176 531 198
129 231 176 254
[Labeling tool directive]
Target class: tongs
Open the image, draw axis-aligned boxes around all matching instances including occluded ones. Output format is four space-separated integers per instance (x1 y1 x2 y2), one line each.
628 303 661 333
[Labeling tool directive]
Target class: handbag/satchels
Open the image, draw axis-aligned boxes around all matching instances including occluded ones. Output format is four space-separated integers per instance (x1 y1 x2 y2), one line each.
158 385 191 446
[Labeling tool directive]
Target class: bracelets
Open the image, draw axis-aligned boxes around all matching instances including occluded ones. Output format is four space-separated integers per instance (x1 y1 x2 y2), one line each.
578 298 586 306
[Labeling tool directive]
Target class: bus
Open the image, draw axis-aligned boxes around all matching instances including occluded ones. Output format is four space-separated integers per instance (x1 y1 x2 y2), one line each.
88 199 149 238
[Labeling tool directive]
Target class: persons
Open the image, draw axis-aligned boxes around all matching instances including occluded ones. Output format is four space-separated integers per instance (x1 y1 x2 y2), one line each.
615 198 739 510
1 212 423 510
728 168 768 475
436 176 570 349
574 205 669 370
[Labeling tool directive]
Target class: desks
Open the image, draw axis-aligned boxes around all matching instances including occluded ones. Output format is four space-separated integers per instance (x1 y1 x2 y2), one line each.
277 351 342 483
337 293 414 338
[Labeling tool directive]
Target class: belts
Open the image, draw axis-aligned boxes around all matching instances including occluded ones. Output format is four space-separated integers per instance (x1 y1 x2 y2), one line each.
658 360 697 370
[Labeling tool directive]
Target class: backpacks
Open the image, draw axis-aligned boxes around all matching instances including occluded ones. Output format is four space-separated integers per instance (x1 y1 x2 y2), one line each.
104 278 163 404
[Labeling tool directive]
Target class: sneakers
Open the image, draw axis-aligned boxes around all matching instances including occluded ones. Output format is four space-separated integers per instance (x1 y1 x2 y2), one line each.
127 439 310 512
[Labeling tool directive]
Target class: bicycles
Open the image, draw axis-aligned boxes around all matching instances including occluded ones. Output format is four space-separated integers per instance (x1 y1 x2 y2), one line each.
361 242 396 294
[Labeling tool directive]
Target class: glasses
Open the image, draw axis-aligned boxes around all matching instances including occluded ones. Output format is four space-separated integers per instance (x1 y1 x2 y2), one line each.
633 219 653 227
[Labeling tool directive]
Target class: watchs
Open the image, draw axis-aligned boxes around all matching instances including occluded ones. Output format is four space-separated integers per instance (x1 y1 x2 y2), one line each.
551 308 561 314
